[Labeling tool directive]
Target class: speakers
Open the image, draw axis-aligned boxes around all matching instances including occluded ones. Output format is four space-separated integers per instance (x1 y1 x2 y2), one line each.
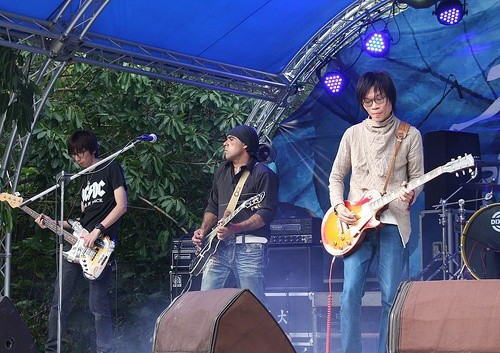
171 271 203 303
265 244 381 292
388 278 500 353
0 296 37 353
151 288 296 353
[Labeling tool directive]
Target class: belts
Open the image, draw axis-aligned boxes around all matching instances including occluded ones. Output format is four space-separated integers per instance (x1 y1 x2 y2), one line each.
224 234 268 246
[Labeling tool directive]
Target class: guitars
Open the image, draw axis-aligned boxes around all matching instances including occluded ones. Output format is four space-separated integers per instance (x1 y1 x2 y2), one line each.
320 153 475 258
188 190 266 278
0 192 116 283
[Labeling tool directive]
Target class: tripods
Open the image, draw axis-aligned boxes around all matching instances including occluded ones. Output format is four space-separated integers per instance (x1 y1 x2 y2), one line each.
413 165 494 282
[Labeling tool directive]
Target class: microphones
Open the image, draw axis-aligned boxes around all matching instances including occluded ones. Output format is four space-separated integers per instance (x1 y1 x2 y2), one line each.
136 134 158 144
452 76 463 98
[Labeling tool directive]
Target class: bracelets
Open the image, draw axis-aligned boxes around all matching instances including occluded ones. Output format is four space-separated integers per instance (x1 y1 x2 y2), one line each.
407 190 415 210
335 204 339 214
234 224 239 234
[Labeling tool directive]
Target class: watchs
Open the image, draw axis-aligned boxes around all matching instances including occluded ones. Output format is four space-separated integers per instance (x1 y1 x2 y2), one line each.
95 223 104 233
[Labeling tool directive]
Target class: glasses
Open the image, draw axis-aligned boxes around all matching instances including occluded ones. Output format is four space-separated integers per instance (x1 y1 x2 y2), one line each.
69 149 88 159
361 95 387 107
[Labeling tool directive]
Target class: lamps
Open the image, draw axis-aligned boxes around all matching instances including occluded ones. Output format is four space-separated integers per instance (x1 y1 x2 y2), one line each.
365 30 391 58
433 1 469 25
322 69 349 96
254 143 276 164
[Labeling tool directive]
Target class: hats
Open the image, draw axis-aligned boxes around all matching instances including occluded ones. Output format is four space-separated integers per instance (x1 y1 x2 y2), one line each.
226 125 259 152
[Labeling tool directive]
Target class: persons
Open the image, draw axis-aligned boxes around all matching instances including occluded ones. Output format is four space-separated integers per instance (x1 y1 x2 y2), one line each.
191 125 278 308
34 129 128 353
329 71 424 353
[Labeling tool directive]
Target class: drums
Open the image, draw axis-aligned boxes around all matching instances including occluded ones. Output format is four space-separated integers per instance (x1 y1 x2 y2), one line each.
460 202 500 280
